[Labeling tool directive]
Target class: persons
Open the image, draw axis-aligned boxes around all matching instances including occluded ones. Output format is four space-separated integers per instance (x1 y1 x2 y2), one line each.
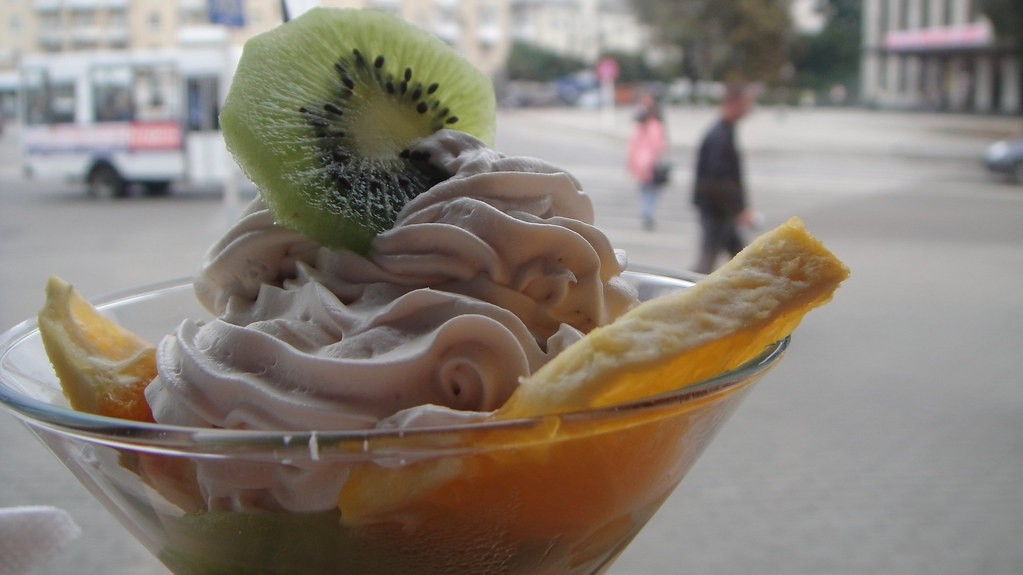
631 83 677 226
693 91 749 276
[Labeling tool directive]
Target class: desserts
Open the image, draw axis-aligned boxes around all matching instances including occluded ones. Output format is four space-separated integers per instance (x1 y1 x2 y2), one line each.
33 2 849 575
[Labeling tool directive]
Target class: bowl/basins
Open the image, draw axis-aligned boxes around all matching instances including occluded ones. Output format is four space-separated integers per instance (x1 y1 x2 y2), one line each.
0 262 791 575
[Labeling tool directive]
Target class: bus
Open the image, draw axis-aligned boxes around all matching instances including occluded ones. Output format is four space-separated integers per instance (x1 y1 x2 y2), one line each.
0 48 248 200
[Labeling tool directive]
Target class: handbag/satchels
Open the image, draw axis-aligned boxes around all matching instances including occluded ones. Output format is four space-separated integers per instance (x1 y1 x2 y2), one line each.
655 159 669 184
